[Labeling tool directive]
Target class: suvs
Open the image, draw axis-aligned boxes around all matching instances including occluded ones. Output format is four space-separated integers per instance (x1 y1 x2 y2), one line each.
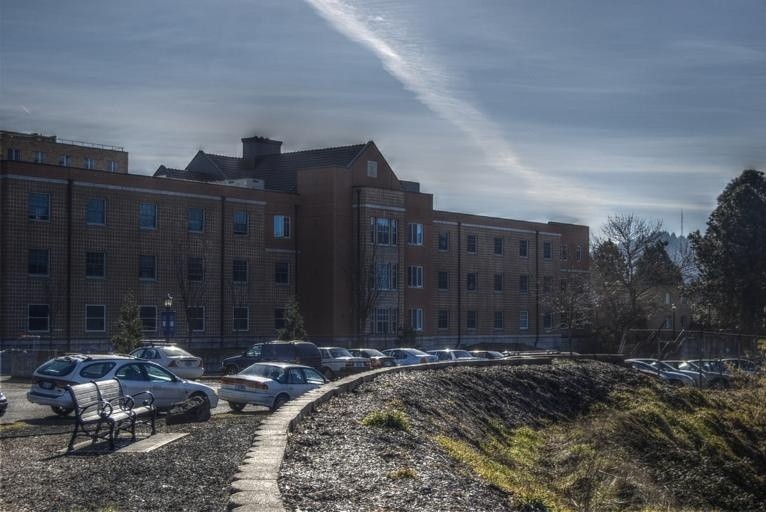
221 342 321 377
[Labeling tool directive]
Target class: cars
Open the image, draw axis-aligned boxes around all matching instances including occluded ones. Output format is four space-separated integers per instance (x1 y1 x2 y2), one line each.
317 346 396 381
217 361 332 415
624 358 765 390
128 346 205 382
383 347 518 366
27 355 219 422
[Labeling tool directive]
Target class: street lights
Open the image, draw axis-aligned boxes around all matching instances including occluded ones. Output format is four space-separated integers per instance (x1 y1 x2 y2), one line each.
164 293 173 346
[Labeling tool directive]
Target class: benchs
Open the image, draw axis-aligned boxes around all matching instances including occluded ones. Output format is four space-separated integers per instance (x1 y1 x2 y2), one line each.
66 377 157 451
